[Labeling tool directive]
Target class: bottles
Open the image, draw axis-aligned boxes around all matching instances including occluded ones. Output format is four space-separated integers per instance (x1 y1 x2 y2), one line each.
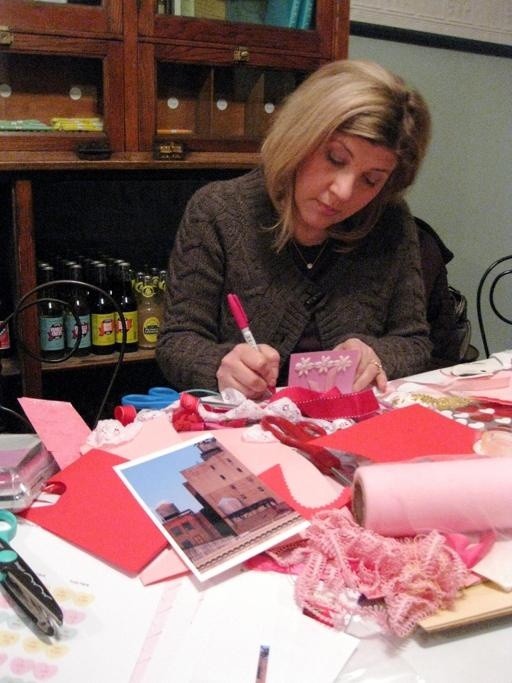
0 287 16 360
36 249 168 361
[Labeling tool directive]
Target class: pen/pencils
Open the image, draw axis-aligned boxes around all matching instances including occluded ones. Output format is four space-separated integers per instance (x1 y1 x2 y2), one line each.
227 293 276 396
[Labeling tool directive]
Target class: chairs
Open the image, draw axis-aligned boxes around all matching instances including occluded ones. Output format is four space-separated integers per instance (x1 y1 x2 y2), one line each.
0 279 129 429
477 253 512 357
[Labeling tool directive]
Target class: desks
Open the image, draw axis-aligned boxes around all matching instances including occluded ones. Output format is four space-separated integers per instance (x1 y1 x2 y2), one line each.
0 349 512 683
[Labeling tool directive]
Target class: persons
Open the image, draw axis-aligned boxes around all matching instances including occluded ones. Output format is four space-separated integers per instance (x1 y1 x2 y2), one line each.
156 58 435 401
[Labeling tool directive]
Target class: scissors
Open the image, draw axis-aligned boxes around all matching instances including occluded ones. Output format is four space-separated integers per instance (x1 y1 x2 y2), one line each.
0 509 63 636
262 415 353 485
121 386 239 411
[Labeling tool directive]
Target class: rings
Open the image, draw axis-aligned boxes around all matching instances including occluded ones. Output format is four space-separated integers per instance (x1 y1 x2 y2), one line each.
368 359 382 374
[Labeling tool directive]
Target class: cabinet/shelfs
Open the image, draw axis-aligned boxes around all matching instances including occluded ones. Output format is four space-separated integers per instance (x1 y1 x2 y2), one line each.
0 0 351 432
